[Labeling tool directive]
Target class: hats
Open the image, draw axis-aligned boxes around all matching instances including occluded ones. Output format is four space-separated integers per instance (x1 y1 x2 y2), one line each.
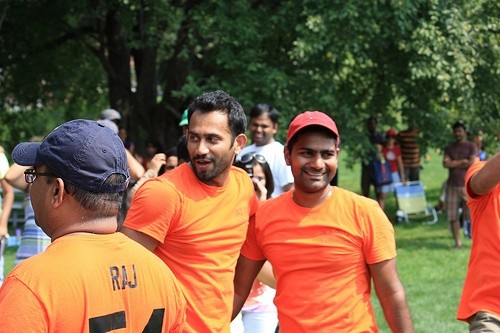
179 109 190 127
386 129 397 136
287 110 340 147
99 108 121 123
11 119 130 193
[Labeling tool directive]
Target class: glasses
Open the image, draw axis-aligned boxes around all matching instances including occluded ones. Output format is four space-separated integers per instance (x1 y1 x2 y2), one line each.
24 169 71 194
239 152 267 166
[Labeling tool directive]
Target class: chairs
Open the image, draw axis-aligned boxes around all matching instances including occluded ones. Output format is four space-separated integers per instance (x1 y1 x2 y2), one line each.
393 181 437 225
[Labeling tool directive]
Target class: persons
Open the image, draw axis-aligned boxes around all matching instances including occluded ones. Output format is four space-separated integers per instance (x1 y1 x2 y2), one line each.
380 129 405 210
125 141 190 212
118 128 134 154
98 108 145 180
5 163 51 267
146 142 165 176
361 116 385 212
179 108 189 141
397 127 422 186
0 119 186 333
232 152 279 333
462 131 487 238
0 151 15 287
444 123 476 248
237 103 294 198
120 89 277 333
456 152 500 333
230 110 414 333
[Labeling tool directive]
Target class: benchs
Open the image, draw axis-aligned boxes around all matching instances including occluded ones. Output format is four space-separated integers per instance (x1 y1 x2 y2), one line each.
0 188 25 228
